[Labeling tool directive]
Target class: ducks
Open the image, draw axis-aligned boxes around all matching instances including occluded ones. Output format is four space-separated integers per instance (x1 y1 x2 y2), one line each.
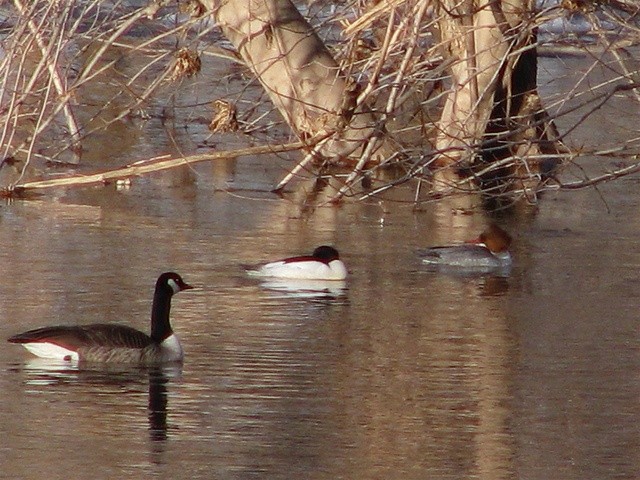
6 271 194 364
410 224 512 268
237 246 348 281
